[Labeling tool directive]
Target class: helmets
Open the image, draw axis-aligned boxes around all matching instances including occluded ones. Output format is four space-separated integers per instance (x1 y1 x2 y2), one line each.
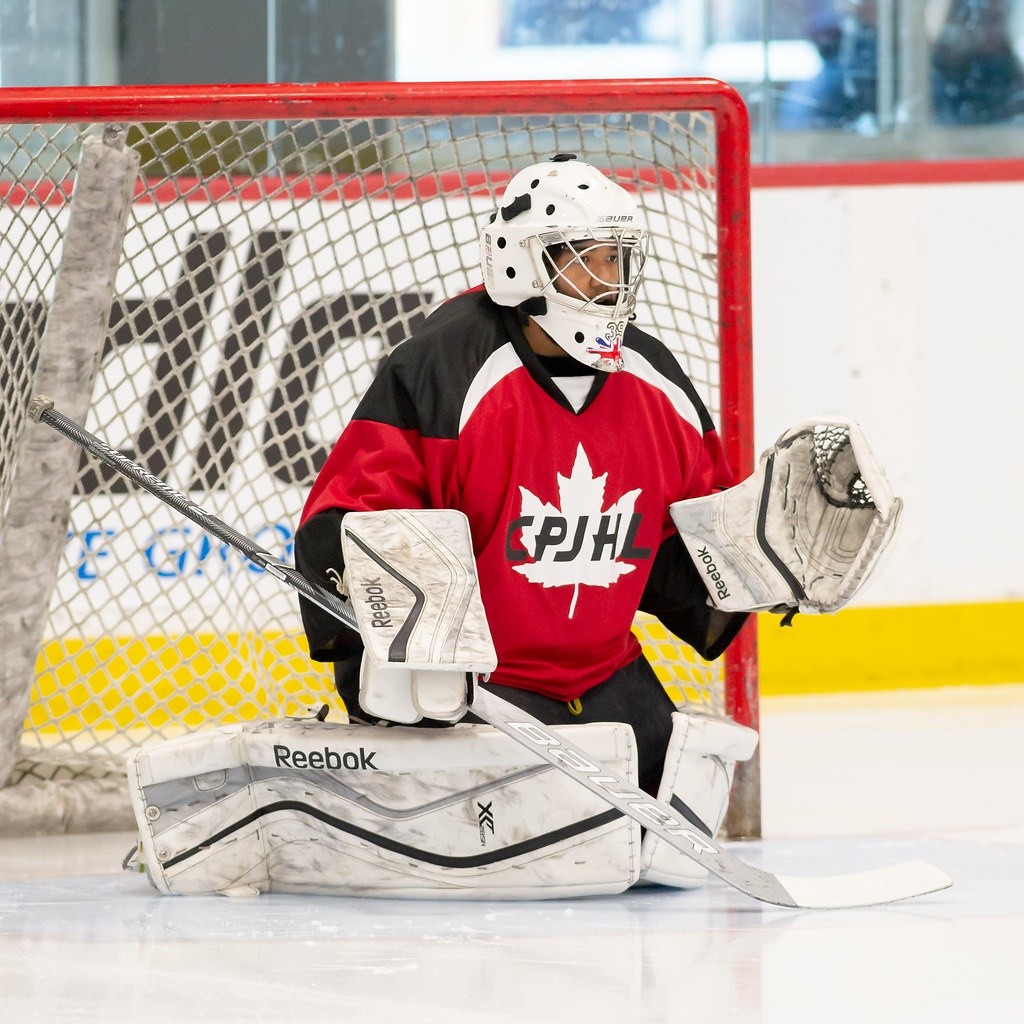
478 153 649 373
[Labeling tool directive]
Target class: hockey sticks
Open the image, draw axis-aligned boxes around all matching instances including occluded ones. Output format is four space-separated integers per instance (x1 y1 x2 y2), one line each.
25 394 957 913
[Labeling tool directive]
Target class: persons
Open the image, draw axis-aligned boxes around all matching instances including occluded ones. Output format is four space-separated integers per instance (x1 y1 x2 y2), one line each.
126 152 903 901
787 1 1024 128
